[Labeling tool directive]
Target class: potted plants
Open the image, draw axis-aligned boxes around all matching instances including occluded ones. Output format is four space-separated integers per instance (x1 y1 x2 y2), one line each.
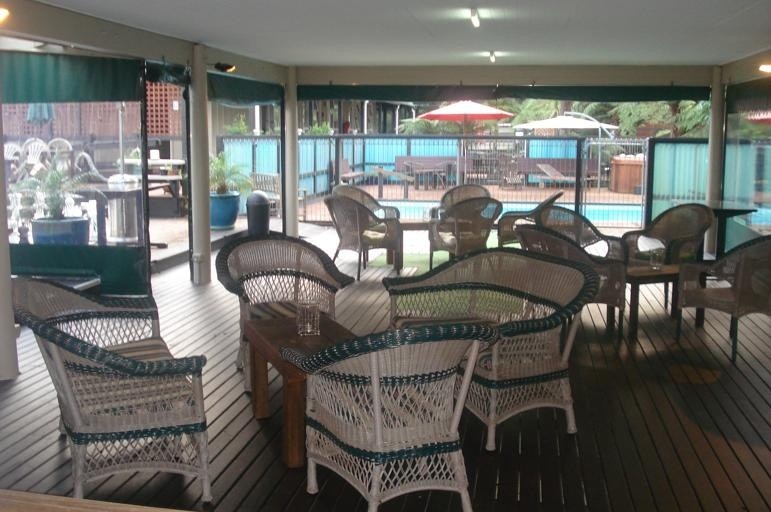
210 152 254 231
8 148 107 245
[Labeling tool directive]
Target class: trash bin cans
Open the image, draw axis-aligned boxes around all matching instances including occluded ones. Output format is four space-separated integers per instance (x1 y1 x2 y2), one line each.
246 190 270 236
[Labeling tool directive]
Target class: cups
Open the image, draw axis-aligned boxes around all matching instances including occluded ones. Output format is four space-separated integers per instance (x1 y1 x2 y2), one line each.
649 250 665 270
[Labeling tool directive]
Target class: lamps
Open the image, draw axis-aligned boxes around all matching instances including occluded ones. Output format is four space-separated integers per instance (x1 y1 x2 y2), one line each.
490 51 495 62
209 62 235 73
470 7 479 28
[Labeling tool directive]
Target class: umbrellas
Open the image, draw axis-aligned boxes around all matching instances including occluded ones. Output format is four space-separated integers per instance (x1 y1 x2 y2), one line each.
514 111 621 194
415 99 514 132
27 102 54 128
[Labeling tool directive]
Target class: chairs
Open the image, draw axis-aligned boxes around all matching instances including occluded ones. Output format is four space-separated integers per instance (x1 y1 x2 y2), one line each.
435 160 456 176
536 163 598 187
405 161 424 176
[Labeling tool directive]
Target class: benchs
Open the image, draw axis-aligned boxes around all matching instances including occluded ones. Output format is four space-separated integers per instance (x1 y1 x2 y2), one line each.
332 158 363 185
395 156 474 173
251 173 306 221
138 175 182 196
516 158 598 187
